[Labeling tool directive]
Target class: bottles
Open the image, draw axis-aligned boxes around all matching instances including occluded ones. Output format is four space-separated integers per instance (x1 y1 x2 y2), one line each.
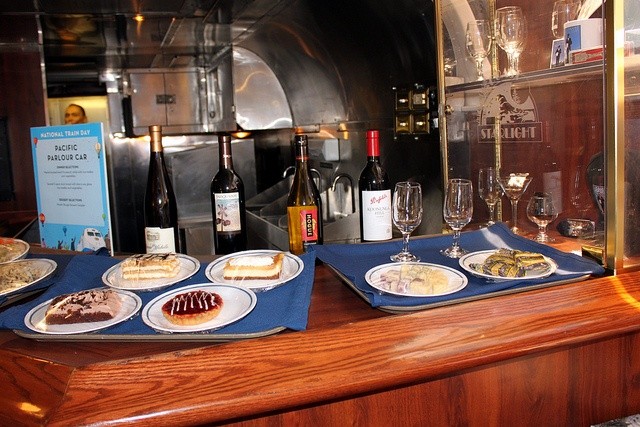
358 129 393 244
541 121 564 218
142 124 180 254
210 133 246 255
286 134 323 256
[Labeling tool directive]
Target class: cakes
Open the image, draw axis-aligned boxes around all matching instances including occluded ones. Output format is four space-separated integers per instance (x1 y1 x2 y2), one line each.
222 252 284 282
514 251 550 276
46 289 121 324
485 248 515 264
162 291 223 326
121 253 182 281
469 262 525 278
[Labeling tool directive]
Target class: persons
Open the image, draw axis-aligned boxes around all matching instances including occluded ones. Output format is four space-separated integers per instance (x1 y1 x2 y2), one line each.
64 104 87 124
566 33 572 62
555 45 561 65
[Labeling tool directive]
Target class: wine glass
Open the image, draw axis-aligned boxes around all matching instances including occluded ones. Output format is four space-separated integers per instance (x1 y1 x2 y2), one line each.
439 178 474 259
525 190 559 242
466 18 492 82
389 181 424 263
497 172 534 236
569 173 593 219
477 167 505 229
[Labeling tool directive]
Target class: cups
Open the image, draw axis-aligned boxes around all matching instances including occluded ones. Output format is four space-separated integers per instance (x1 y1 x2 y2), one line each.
551 0 583 39
494 5 528 77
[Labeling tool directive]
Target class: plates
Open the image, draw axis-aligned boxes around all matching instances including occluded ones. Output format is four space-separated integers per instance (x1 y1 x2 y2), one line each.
102 254 201 293
1 257 58 296
24 288 142 335
458 250 558 283
141 283 257 334
0 237 31 264
204 249 304 293
364 262 469 298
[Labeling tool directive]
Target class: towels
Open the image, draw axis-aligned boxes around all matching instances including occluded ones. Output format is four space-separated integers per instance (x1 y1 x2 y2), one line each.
308 221 606 307
0 253 317 333
0 252 75 306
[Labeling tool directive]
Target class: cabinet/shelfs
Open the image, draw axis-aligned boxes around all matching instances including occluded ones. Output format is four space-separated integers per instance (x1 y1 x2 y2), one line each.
434 0 640 275
